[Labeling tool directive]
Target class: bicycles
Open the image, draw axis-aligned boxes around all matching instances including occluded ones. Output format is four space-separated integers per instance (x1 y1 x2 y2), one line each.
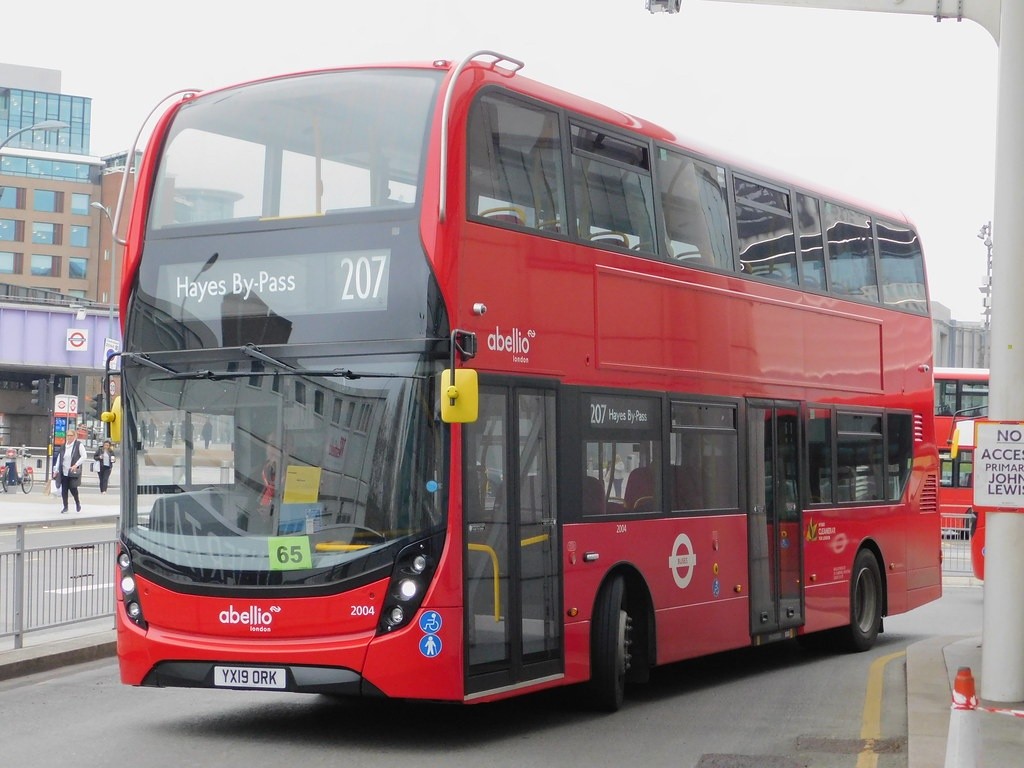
1 448 34 494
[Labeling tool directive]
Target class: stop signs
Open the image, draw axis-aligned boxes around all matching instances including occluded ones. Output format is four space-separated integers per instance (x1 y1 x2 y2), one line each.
27 467 33 474
6 449 17 458
0 466 6 474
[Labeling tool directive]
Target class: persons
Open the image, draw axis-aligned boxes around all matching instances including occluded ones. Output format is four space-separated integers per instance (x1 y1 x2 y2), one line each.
167 420 174 448
139 419 158 449
53 429 88 513
232 427 294 515
614 454 626 498
93 440 116 495
201 418 213 449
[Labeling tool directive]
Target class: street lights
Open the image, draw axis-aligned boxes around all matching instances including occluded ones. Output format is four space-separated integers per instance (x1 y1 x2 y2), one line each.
90 201 117 447
645 1 1024 722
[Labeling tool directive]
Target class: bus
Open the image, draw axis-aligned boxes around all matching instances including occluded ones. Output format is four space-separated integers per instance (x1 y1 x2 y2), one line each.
101 53 944 703
931 368 991 539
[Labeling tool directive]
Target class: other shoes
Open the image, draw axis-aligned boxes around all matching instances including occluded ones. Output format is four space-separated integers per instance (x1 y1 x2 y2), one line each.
61 507 68 513
101 491 106 494
76 501 81 512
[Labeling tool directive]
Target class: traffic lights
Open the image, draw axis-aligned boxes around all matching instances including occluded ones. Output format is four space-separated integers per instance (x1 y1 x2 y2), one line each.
91 394 102 421
31 378 47 409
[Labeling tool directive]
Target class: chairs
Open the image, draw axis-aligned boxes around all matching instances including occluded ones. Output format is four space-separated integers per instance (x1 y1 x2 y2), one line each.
479 206 865 296
495 466 703 515
938 404 952 416
972 405 981 416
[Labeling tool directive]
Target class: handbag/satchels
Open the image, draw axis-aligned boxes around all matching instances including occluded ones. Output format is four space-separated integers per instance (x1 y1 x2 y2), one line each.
51 479 62 497
93 460 100 472
69 468 80 478
